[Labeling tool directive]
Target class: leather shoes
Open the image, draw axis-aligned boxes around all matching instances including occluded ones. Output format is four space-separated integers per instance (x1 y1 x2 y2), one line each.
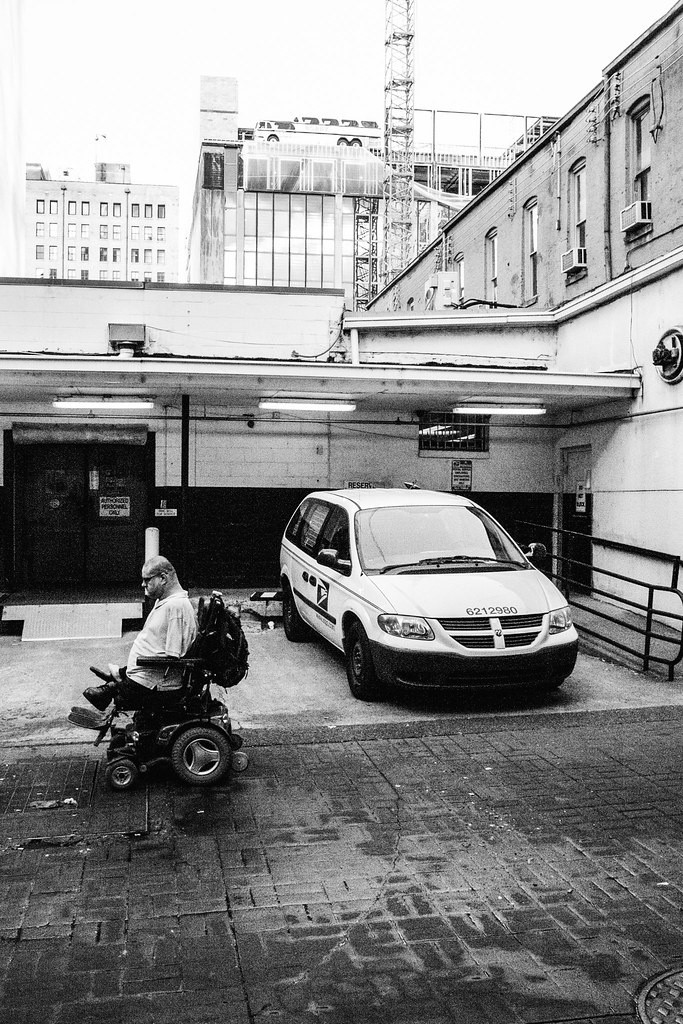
83 682 117 712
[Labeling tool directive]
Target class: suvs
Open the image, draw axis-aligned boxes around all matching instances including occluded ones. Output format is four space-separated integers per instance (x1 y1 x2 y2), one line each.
280 488 578 702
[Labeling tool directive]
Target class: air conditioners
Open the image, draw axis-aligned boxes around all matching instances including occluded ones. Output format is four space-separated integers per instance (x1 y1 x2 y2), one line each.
620 201 653 232
561 247 587 273
424 271 460 311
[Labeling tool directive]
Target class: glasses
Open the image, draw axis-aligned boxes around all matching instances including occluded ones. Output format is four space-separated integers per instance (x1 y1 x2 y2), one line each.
142 573 161 583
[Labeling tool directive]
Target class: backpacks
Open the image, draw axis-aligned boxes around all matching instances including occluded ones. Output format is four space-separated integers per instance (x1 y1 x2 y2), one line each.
196 595 250 695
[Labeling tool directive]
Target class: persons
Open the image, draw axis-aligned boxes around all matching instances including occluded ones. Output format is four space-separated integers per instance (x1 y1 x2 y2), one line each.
82 555 201 712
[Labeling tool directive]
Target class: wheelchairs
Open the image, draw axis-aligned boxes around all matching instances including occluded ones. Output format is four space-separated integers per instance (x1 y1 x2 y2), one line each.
67 591 250 790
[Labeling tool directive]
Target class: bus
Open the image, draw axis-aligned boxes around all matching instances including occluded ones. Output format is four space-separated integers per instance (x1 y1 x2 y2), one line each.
252 117 381 152
